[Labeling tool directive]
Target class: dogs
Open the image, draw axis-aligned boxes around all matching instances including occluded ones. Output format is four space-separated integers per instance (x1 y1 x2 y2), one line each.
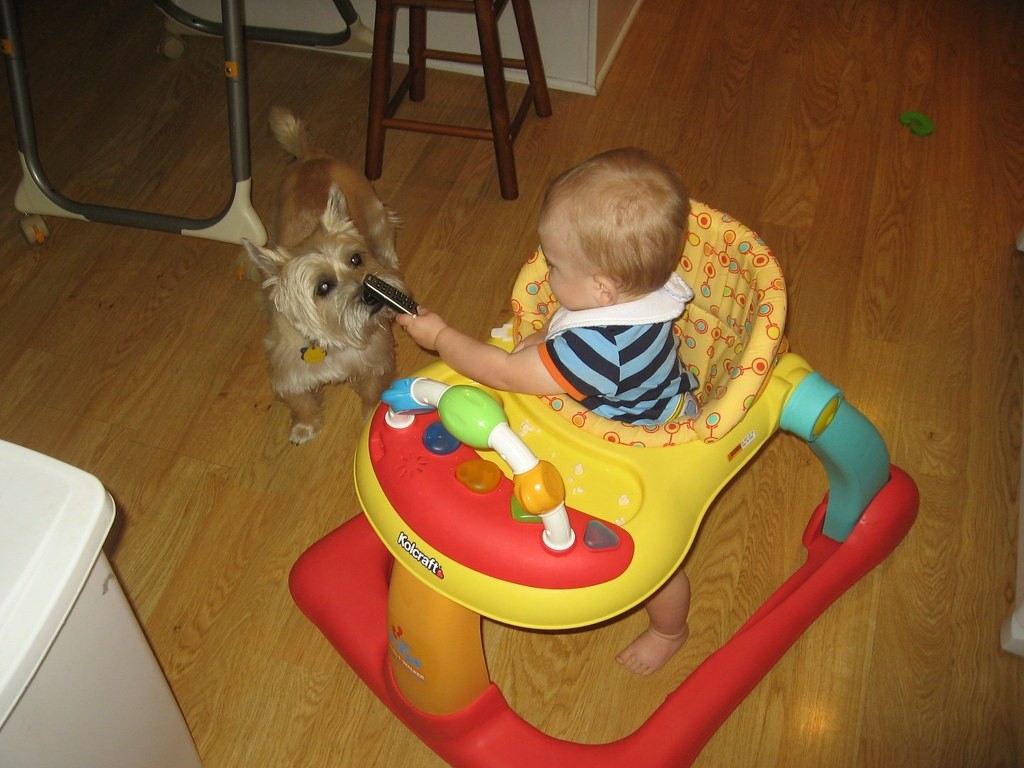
239 106 410 447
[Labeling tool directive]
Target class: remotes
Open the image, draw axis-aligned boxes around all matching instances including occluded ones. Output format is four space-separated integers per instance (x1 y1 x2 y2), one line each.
363 274 419 318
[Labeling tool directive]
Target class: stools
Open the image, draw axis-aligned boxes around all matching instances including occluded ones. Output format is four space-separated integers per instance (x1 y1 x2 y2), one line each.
360 0 553 202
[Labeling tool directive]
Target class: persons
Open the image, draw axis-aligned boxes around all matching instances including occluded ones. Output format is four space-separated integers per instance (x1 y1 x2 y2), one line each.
396 146 712 676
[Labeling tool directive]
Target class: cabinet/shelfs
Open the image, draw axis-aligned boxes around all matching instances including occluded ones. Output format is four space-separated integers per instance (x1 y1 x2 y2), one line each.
163 0 644 99
